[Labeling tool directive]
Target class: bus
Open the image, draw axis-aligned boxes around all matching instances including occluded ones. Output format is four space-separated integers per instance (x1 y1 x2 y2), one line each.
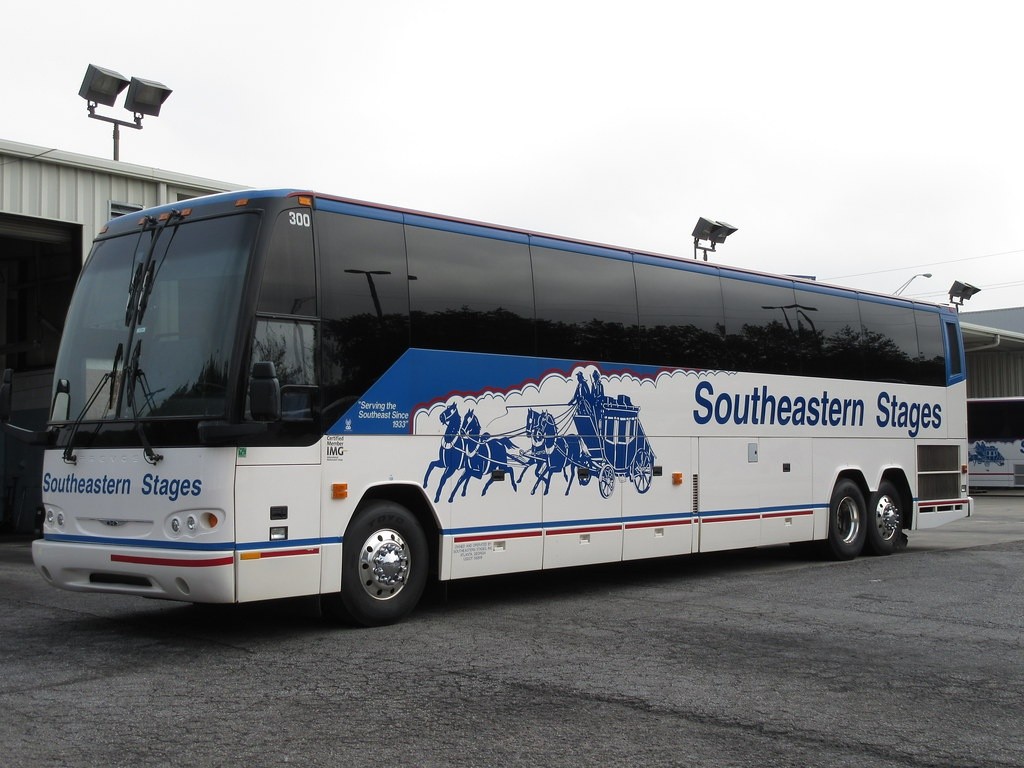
966 396 1024 491
1 190 975 627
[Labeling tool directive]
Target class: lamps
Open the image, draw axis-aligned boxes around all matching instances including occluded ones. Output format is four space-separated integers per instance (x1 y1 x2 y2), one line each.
78 64 173 165
948 281 981 315
691 218 737 264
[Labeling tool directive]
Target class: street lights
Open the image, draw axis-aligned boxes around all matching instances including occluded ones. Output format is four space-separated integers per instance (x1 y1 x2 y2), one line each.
894 274 932 298
691 215 738 265
78 63 174 160
947 282 981 317
